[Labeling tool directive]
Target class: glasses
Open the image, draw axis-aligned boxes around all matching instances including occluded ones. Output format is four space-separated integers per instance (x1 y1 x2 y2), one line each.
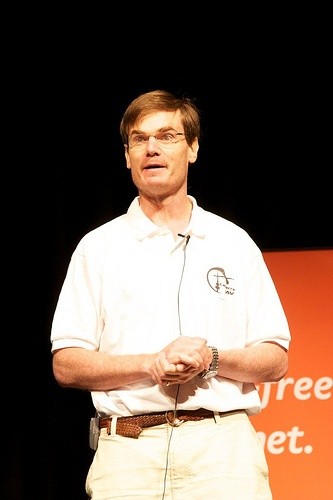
126 131 190 146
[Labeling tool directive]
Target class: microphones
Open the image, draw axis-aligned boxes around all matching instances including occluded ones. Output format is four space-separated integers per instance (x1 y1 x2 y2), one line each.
178 233 190 245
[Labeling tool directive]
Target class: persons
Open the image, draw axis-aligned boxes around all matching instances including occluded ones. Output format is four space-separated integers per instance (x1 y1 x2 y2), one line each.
49 89 291 500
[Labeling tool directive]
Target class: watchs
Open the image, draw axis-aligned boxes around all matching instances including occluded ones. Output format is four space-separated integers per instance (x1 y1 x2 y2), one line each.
198 346 219 380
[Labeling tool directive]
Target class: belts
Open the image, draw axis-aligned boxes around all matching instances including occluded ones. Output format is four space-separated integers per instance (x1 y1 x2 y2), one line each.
93 409 249 437
